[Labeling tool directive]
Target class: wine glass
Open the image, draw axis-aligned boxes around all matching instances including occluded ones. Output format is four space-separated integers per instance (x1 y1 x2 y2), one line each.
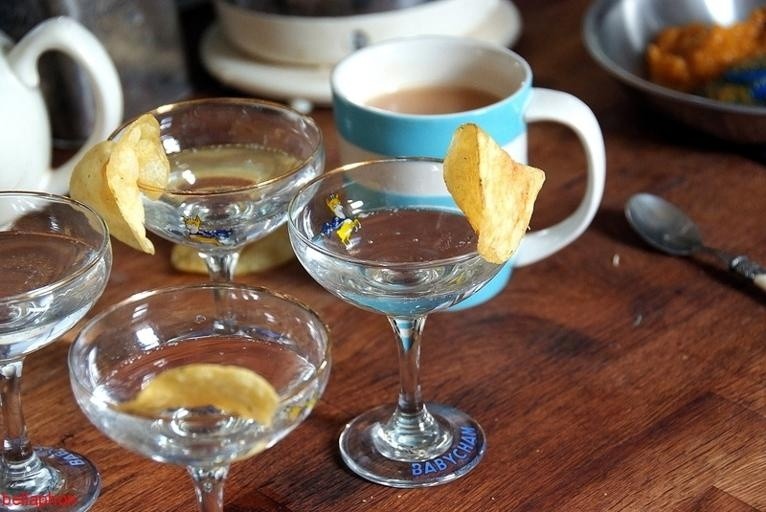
0 183 116 511
98 95 327 358
285 154 532 490
65 278 333 511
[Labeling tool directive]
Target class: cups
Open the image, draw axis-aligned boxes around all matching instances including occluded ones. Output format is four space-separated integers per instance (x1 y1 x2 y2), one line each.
208 0 510 67
0 16 125 239
330 29 608 314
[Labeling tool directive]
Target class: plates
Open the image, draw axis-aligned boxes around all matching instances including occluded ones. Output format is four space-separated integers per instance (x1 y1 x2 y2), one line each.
579 0 766 144
198 0 525 111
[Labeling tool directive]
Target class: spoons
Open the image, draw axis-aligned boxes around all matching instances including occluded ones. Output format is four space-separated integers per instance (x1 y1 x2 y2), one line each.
624 190 766 294
